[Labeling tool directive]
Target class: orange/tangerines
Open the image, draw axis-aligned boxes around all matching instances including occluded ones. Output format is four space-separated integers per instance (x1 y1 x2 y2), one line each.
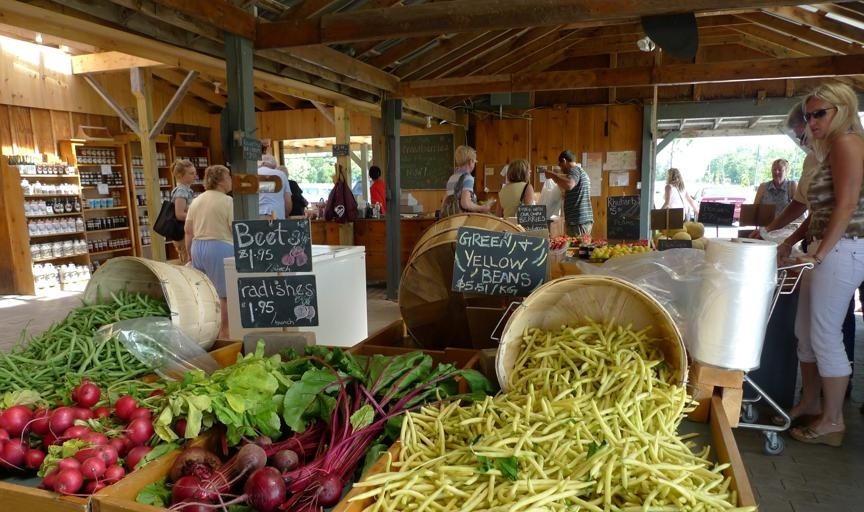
590 243 654 262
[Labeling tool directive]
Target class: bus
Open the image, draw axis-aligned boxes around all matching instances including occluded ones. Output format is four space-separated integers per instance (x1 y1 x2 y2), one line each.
296 182 335 206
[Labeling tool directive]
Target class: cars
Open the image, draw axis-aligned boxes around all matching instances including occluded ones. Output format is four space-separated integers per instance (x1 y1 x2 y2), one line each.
654 180 756 222
352 180 371 198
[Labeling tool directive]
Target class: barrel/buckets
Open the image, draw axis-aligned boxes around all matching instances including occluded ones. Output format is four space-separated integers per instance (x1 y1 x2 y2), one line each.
398 212 525 349
490 274 700 420
82 255 222 356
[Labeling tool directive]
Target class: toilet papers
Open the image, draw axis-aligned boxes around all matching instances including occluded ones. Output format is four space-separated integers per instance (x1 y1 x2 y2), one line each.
576 235 779 373
256 181 276 193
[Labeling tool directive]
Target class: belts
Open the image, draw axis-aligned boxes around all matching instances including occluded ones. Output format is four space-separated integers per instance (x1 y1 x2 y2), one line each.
805 233 864 245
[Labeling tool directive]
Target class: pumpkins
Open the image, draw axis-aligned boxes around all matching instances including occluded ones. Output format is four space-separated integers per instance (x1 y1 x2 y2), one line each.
653 220 709 252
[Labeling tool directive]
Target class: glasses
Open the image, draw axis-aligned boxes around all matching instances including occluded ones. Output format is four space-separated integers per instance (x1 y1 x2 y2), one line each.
803 107 836 122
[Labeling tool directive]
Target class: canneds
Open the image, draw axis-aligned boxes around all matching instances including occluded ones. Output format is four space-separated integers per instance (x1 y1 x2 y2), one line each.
132 156 143 166
138 216 148 225
76 148 132 253
134 171 168 186
176 156 208 181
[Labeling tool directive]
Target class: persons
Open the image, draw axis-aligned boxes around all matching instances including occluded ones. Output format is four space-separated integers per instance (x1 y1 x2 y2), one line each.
748 102 855 400
183 165 234 340
752 158 798 215
544 150 595 237
660 168 699 223
495 159 534 217
446 145 490 214
771 83 864 447
257 154 293 220
169 158 197 264
276 166 309 215
369 166 386 213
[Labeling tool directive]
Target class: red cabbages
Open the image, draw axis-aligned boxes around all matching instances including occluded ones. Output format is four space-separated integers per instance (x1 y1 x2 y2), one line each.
168 434 343 512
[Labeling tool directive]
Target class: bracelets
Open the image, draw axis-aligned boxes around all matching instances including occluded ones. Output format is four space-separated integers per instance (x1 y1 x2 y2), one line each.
813 255 822 264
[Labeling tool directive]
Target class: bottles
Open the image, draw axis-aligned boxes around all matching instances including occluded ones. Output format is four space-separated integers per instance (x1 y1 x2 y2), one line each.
85 237 132 253
26 216 84 235
28 238 87 262
85 215 129 232
19 178 78 196
23 196 82 216
317 198 325 218
374 201 380 220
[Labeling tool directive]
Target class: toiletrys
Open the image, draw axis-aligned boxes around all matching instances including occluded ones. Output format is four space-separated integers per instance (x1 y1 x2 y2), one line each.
9 147 209 297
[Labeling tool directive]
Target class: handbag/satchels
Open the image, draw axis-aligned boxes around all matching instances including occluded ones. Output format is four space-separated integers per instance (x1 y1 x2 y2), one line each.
441 194 465 218
153 200 187 240
324 181 359 223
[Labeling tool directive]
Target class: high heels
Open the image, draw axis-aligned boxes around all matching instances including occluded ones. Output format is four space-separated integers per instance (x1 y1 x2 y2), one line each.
771 405 824 426
788 416 846 447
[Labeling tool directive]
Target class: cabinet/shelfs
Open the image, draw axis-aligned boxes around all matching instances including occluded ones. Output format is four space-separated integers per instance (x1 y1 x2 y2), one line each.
116 135 175 257
59 138 137 258
0 153 90 298
172 132 210 184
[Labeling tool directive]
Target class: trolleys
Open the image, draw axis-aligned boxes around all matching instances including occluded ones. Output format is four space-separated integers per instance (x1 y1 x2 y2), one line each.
737 260 813 457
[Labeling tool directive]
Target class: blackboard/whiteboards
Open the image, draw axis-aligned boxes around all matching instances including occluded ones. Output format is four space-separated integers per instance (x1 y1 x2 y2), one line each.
400 133 455 189
697 201 735 226
517 205 547 227
233 217 311 272
332 144 348 156
607 196 641 239
237 274 318 327
243 136 262 162
658 240 692 250
451 227 547 295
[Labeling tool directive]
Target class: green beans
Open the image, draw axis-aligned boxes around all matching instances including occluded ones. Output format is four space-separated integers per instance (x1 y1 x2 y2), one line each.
0 284 171 450
349 314 761 512
